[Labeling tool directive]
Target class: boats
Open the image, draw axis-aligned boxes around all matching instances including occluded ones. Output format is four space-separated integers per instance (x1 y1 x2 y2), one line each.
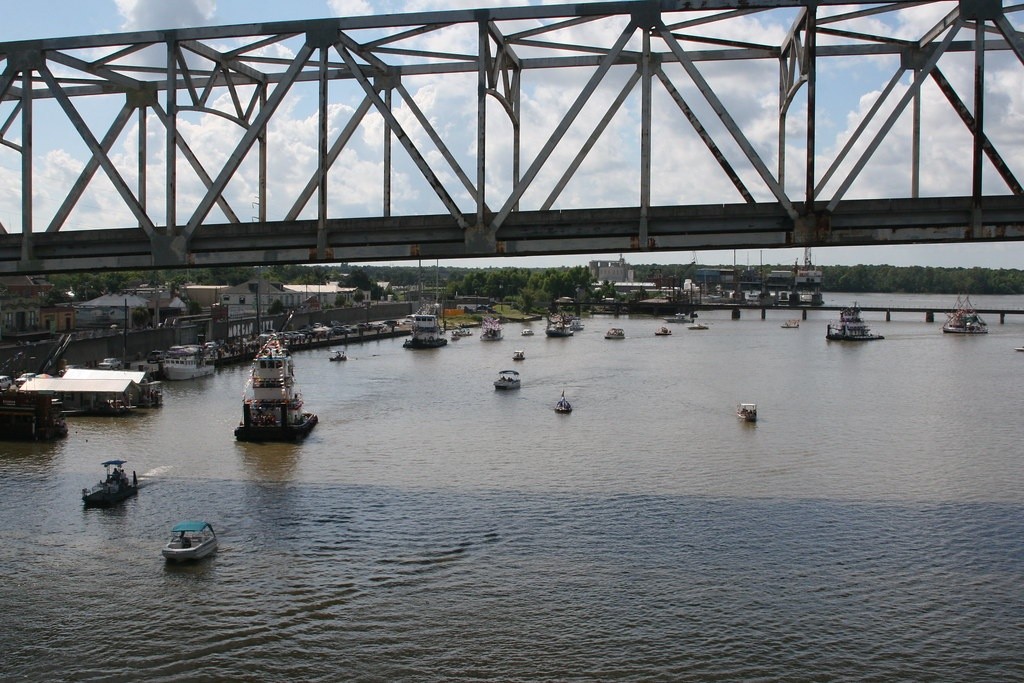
163 358 214 381
402 315 448 348
943 293 989 333
493 370 521 389
521 329 535 336
329 351 347 360
161 519 218 561
512 351 525 360
655 326 672 335
439 328 473 340
544 313 584 337
480 316 504 340
736 402 758 422
603 327 624 338
555 397 572 413
780 321 800 328
663 314 690 323
825 303 884 340
234 333 319 442
686 324 709 330
81 457 136 504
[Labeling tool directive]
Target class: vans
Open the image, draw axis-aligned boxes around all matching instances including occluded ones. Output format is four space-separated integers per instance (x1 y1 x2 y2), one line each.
0 376 12 390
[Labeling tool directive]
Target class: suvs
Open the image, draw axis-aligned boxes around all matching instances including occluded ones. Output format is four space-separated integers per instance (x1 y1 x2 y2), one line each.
15 372 36 387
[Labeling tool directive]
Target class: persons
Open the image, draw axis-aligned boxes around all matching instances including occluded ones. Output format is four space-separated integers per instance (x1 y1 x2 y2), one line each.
59 418 64 426
120 470 126 480
180 531 184 541
210 343 257 357
113 468 120 480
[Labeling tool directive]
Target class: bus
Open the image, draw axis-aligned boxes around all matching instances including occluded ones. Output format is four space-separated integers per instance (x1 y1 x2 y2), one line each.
456 297 490 309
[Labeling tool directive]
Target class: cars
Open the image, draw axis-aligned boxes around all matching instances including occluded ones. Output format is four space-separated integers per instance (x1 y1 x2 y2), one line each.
59 365 85 377
146 342 221 363
98 358 122 369
231 319 415 353
